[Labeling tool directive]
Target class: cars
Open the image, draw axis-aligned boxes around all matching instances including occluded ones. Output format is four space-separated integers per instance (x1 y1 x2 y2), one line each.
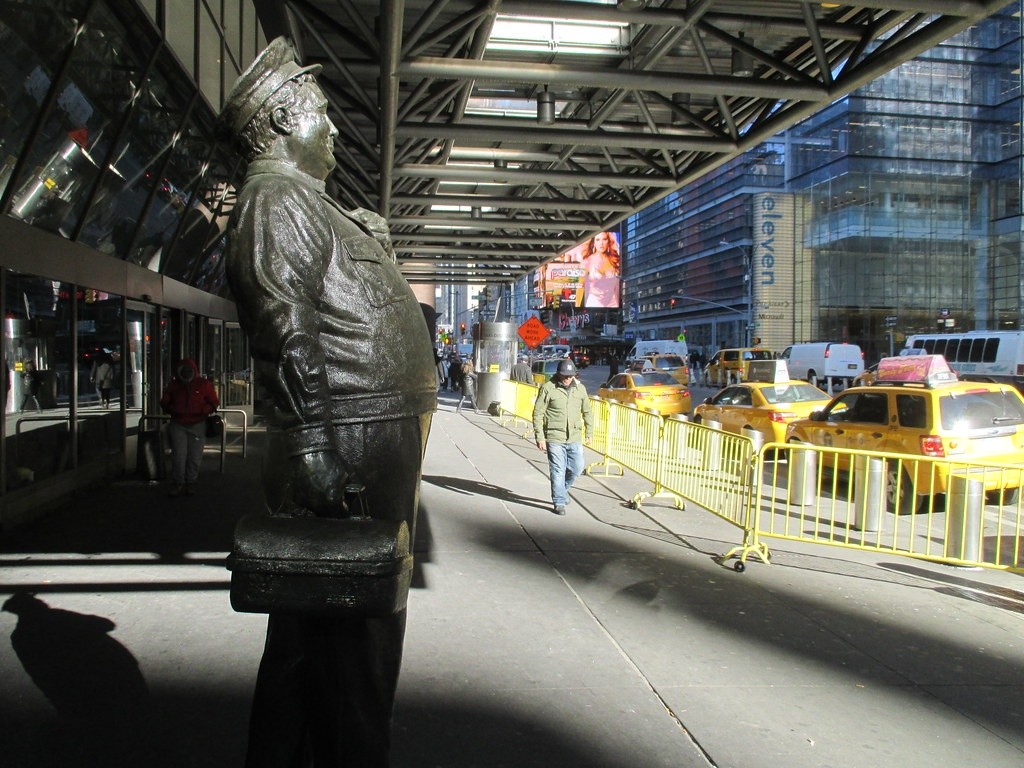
584 356 590 367
702 347 772 386
568 352 586 369
625 352 689 386
624 339 689 369
597 368 691 425
692 379 847 462
530 358 579 382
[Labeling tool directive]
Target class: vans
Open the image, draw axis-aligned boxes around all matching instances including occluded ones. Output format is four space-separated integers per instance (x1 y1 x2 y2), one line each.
783 352 1023 517
777 342 867 390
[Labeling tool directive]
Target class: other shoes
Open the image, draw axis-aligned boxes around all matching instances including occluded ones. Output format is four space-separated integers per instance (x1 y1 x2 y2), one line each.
555 505 566 515
185 483 195 495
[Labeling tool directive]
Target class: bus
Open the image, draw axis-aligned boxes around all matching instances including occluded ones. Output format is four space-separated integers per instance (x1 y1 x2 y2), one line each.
899 329 1024 396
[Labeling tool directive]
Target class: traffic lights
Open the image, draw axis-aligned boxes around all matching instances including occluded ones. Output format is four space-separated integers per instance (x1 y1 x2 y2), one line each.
460 323 466 335
439 329 443 332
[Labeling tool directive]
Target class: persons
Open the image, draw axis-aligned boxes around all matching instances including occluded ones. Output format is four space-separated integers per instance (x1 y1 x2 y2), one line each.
532 358 594 515
161 359 219 498
220 32 436 768
575 232 620 308
5 350 119 414
432 348 782 415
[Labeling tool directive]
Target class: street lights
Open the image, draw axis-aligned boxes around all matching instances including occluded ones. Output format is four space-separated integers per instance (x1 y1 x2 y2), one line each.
718 241 752 347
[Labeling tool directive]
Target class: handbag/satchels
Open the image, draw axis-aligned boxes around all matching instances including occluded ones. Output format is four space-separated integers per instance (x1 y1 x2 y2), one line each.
96 380 104 388
205 415 223 438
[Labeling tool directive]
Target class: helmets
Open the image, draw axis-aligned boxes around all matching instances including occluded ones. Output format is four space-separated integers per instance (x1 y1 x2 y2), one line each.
558 360 575 376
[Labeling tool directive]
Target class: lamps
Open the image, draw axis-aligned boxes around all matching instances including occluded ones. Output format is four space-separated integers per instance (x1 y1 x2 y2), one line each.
471 206 482 219
494 160 507 183
617 0 646 12
672 93 690 124
732 31 753 77
814 3 844 12
537 85 555 125
